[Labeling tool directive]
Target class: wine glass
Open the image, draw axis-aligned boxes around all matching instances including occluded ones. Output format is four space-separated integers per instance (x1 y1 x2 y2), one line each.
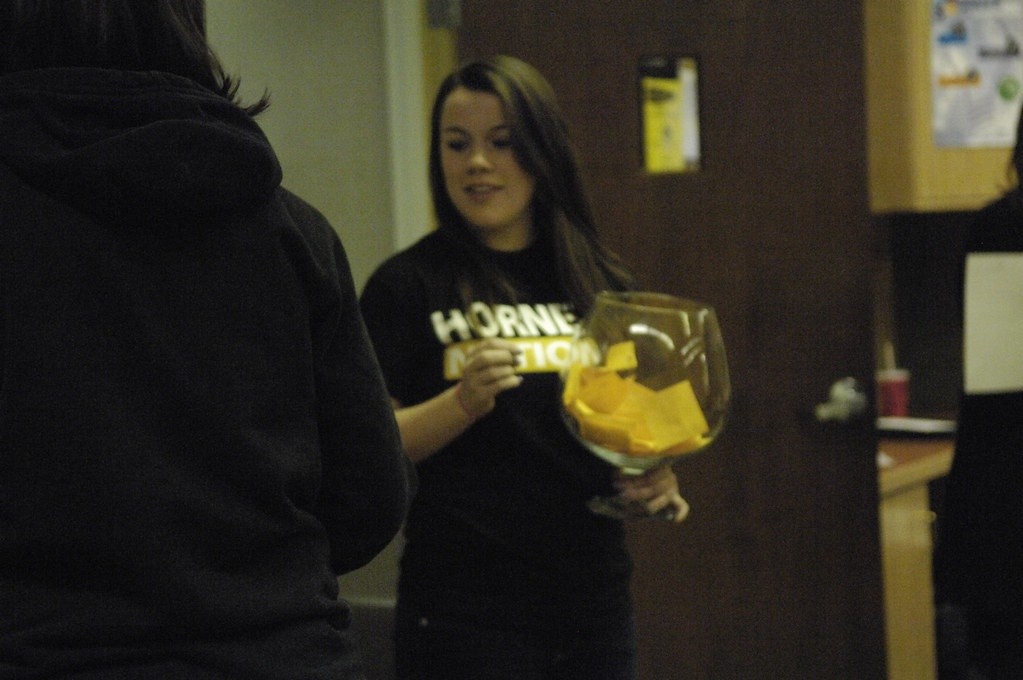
558 290 731 530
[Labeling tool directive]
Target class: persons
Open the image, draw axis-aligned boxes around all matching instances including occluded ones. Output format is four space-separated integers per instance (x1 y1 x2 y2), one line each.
947 102 1022 680
359 56 691 680
0 1 411 680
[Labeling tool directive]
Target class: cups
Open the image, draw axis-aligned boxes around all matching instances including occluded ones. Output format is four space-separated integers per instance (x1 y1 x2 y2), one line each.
876 370 908 418
